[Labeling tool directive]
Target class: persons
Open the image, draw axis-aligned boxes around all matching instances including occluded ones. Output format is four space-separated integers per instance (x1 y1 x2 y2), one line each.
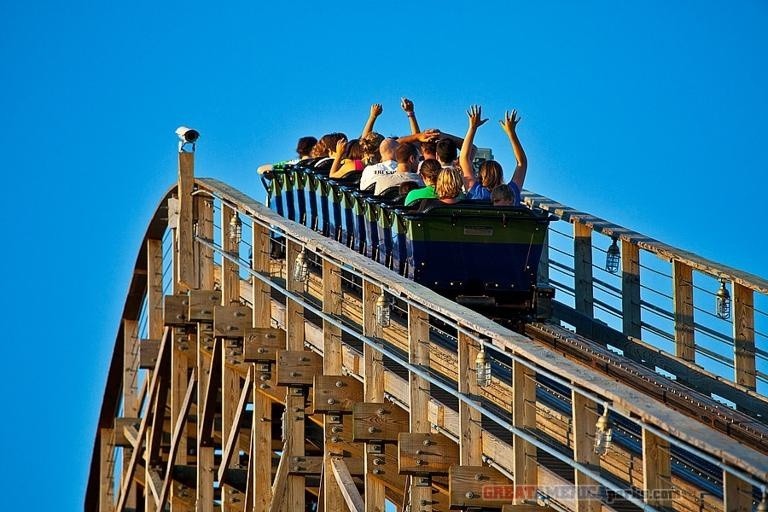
256 96 528 211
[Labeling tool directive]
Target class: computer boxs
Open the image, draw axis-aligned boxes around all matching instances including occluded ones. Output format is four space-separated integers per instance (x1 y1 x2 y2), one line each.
175 126 200 143
